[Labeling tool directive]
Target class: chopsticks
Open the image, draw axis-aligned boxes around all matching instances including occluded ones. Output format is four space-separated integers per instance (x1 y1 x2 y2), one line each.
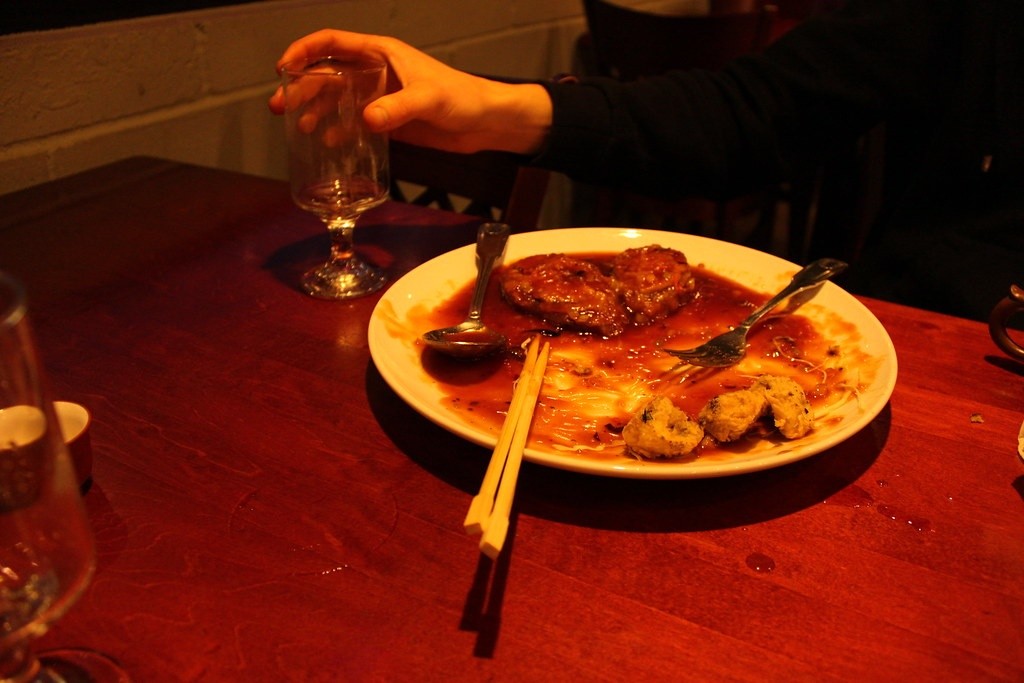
463 328 552 561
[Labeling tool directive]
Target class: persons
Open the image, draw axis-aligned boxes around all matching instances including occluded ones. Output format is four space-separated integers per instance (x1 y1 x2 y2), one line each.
268 0 1024 333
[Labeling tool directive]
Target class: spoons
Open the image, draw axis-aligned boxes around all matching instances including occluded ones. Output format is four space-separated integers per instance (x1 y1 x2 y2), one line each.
414 218 514 361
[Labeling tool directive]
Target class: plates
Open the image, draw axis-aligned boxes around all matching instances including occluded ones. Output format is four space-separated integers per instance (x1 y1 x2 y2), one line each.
366 226 900 482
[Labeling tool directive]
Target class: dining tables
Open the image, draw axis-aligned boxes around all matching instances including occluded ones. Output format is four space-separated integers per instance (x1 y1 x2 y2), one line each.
0 157 1024 683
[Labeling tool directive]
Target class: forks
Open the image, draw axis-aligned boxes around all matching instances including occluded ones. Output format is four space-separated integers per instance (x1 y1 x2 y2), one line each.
656 254 851 371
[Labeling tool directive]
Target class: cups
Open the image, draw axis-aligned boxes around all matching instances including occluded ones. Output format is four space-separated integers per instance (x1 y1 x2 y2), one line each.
0 401 94 505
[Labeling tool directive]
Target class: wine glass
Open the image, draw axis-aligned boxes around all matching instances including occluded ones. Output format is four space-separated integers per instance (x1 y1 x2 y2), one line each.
277 48 391 300
0 301 131 683
